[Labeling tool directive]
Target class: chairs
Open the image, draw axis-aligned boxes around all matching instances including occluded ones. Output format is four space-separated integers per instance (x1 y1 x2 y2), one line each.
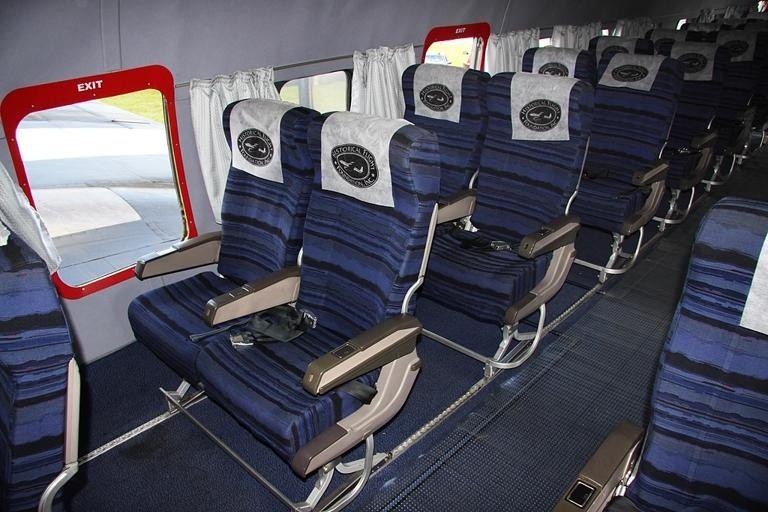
645 6 768 41
650 42 730 232
401 62 491 240
590 35 656 82
126 99 320 414
551 196 767 512
573 52 684 284
701 29 767 168
417 72 595 377
194 110 440 512
524 48 595 81
1 237 80 512
